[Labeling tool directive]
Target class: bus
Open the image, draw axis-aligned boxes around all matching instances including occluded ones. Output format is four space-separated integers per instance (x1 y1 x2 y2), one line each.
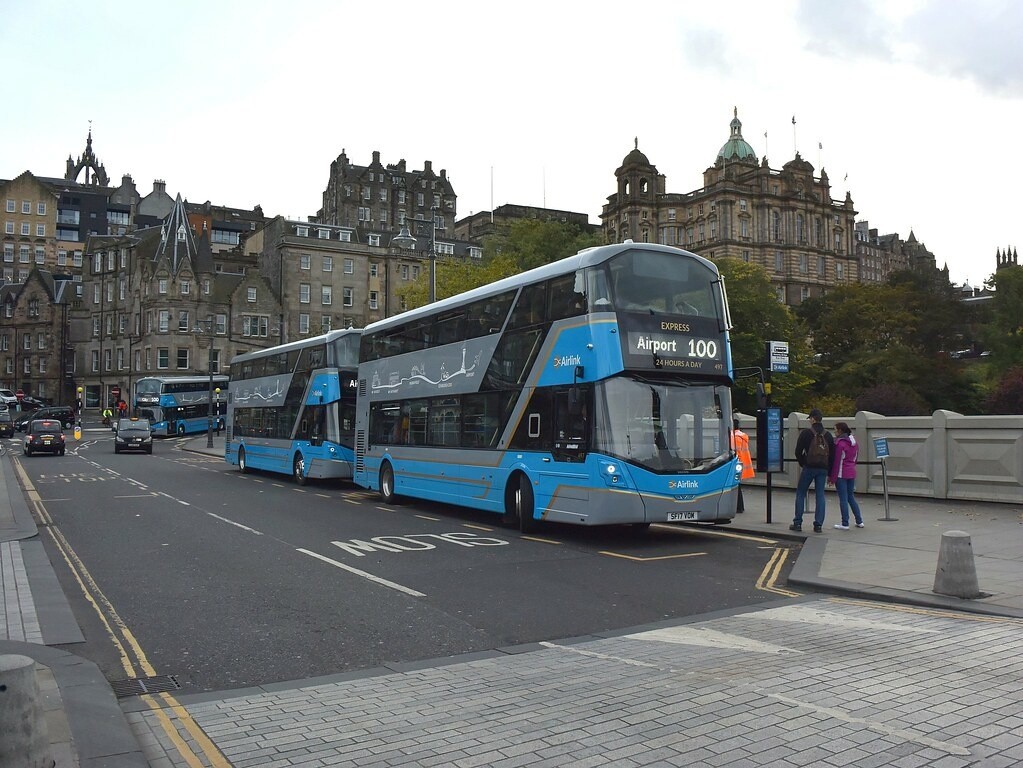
133 374 229 439
224 326 364 486
353 238 767 535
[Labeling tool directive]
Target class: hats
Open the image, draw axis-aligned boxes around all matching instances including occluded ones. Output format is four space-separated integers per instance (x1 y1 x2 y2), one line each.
806 408 821 419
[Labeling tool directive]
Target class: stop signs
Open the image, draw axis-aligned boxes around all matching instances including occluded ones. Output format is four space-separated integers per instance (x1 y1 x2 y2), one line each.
111 387 120 396
15 390 24 399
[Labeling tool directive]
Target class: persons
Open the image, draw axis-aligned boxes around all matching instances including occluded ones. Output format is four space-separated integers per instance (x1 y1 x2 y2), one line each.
829 422 864 530
730 419 755 513
789 409 835 533
102 407 113 421
118 399 127 418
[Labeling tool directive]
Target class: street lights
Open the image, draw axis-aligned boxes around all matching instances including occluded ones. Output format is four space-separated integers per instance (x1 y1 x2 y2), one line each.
190 315 216 448
392 204 438 304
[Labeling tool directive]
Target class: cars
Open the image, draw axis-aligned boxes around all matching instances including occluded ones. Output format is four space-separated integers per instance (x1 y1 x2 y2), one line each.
0 387 51 438
22 419 66 456
111 417 156 455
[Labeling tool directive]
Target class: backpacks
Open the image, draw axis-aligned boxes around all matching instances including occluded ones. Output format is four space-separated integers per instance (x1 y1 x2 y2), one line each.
805 427 830 466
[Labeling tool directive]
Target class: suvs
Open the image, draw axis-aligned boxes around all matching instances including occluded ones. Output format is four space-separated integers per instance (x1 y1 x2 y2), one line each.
14 406 76 434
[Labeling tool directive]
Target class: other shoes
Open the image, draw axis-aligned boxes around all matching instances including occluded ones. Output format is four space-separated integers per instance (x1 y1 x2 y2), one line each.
813 525 822 532
789 524 801 531
834 524 849 529
855 522 864 527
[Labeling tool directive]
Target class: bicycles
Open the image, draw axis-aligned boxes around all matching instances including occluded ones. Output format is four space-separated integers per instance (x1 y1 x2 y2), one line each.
103 415 113 428
